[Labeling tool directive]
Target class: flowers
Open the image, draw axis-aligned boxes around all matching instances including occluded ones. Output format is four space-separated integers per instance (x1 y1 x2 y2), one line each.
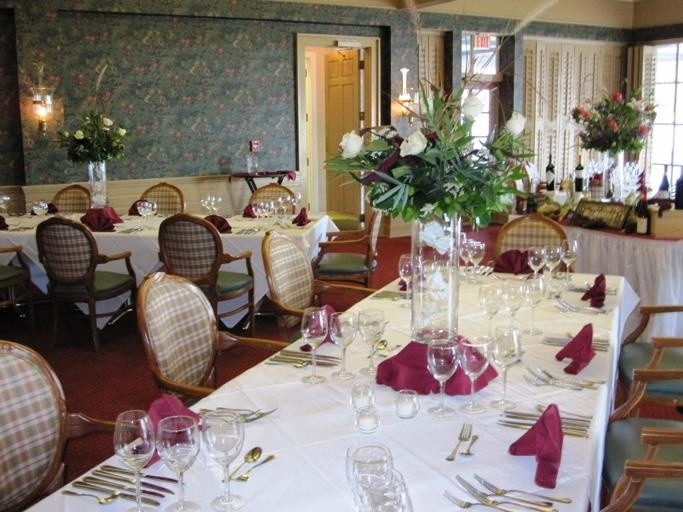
55 92 129 165
562 80 659 163
322 34 542 229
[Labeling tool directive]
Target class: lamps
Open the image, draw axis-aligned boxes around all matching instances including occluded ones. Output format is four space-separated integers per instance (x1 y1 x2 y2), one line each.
399 66 413 118
29 88 55 141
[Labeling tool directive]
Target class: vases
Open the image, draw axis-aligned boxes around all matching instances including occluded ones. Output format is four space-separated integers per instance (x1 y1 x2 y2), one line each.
87 161 107 209
408 215 459 347
602 146 622 201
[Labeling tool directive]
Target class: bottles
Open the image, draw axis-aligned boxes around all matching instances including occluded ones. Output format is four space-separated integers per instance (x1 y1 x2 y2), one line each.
572 154 584 192
634 191 649 236
672 165 683 210
655 164 670 202
545 154 554 192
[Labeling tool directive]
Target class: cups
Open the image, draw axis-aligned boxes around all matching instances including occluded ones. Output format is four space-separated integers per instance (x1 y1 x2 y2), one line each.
395 389 420 420
350 383 376 412
354 407 380 435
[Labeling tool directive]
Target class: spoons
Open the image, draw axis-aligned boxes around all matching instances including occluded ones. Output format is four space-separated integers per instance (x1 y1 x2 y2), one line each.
220 446 261 483
59 489 120 507
362 339 387 358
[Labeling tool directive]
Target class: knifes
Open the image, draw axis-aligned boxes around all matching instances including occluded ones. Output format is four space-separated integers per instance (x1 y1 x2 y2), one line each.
71 481 161 506
82 476 164 498
100 465 178 483
91 469 175 496
264 349 341 370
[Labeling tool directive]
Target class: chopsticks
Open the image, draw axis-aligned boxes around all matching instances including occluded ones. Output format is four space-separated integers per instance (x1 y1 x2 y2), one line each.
496 420 588 438
498 410 590 428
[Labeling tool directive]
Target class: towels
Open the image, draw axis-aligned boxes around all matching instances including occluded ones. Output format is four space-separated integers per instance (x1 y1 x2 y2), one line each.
309 303 336 348
555 320 597 374
493 246 543 275
509 404 566 488
143 392 209 469
397 260 427 290
580 272 606 307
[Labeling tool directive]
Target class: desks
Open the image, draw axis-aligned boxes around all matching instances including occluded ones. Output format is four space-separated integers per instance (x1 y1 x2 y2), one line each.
509 210 682 347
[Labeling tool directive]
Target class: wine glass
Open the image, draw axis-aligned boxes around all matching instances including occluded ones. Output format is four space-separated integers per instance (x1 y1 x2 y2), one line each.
460 239 473 277
490 325 522 411
251 202 259 225
397 253 421 309
32 201 50 225
113 407 155 512
344 439 392 490
501 280 524 332
272 196 281 219
542 245 561 291
199 192 212 216
458 336 492 414
136 199 158 231
291 191 301 220
526 247 544 279
201 409 246 512
155 414 202 512
328 310 356 381
280 194 290 219
354 470 414 512
521 277 546 336
477 279 503 335
559 238 577 287
210 193 223 216
467 241 486 281
298 305 329 385
423 338 460 420
357 309 386 376
258 201 265 225
264 200 274 223
0 193 11 222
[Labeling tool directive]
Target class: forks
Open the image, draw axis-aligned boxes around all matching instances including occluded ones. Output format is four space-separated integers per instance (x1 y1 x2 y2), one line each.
522 363 607 393
442 490 551 509
468 489 517 512
458 435 478 457
538 335 610 353
230 455 274 482
563 283 615 296
200 406 277 424
439 422 472 461
453 475 558 512
472 474 571 504
553 299 611 315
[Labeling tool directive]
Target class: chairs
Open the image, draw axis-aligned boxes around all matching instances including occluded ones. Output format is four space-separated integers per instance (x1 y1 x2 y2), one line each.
264 224 377 341
136 269 294 411
599 304 681 508
494 211 574 274
1 336 153 510
